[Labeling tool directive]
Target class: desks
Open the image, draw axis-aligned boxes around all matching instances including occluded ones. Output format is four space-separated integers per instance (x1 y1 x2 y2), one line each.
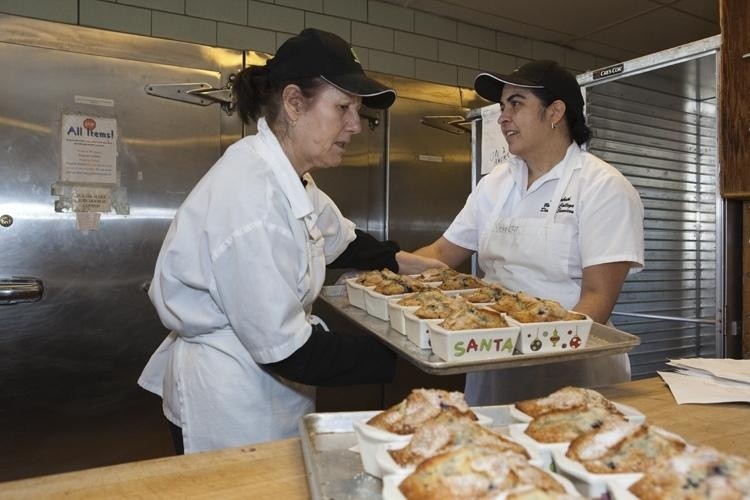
1 374 749 500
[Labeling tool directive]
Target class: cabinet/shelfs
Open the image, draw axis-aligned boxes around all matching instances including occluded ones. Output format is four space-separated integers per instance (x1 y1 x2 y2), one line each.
718 0 750 199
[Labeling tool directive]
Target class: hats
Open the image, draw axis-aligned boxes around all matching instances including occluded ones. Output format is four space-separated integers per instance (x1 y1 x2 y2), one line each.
472 60 583 103
271 27 397 111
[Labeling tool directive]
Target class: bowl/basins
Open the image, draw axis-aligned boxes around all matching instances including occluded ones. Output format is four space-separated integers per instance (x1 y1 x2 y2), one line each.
370 425 547 477
375 464 584 500
507 395 644 427
607 457 749 500
507 416 633 461
350 409 494 479
550 429 688 500
343 276 594 363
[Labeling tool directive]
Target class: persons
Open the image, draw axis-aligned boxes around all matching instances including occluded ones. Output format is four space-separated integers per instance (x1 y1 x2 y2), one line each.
138 25 451 463
402 58 643 406
